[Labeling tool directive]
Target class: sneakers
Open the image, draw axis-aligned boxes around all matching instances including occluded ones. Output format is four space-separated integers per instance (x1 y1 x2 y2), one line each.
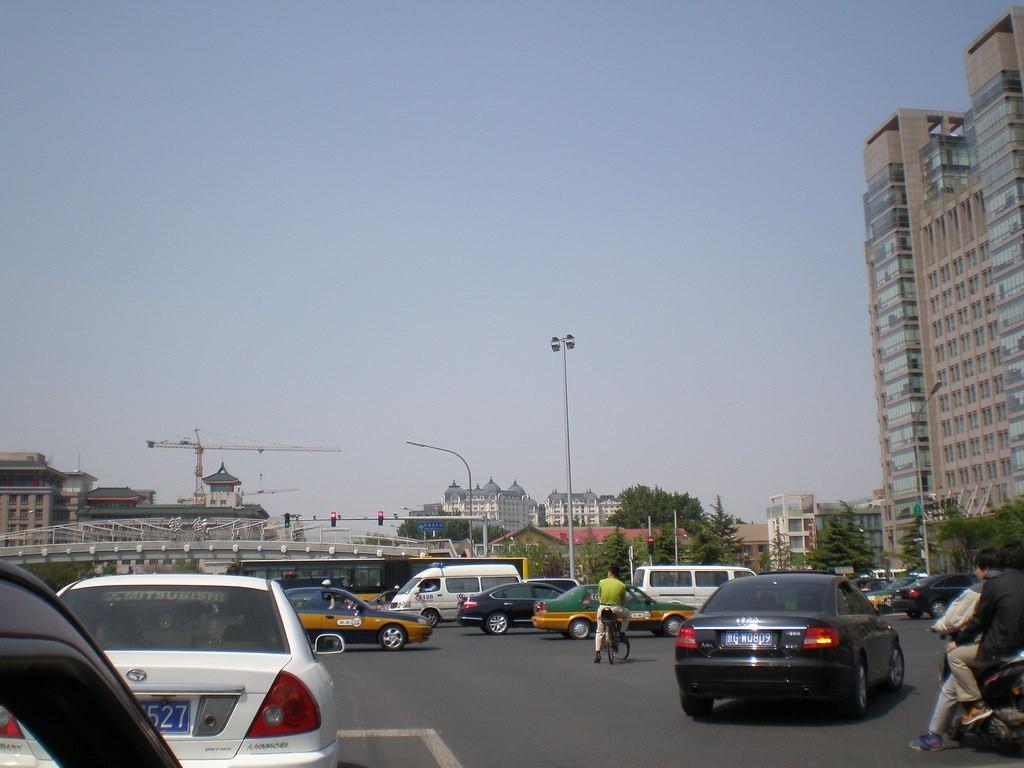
961 703 992 725
909 732 944 750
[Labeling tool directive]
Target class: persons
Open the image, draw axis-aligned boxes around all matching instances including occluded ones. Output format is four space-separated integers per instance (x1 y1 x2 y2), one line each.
593 564 631 664
420 580 439 592
324 592 336 610
909 540 1024 751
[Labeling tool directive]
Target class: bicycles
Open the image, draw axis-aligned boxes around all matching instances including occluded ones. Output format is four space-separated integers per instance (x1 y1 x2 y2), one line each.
601 607 630 665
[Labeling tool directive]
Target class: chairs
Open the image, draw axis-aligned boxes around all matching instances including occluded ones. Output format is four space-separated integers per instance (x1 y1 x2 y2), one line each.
87 599 280 654
734 586 823 612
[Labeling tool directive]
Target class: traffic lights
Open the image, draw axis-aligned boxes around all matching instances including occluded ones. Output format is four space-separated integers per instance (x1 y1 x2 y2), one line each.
648 536 654 556
331 512 336 527
285 513 289 527
378 510 384 526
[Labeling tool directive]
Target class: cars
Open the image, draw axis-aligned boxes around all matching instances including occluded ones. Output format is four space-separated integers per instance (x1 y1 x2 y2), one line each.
457 583 567 636
2 571 340 768
0 554 176 768
851 577 982 619
362 590 398 612
283 586 433 651
675 569 906 718
532 583 698 637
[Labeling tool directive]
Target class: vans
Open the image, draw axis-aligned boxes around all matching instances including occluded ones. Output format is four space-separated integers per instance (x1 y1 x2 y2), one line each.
634 566 759 612
390 565 524 627
521 577 580 593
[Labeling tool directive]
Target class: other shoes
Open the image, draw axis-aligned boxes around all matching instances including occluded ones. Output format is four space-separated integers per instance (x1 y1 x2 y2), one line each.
619 632 626 643
594 655 601 663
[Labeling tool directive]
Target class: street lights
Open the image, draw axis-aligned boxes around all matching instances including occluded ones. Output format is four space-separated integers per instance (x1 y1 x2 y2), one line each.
405 440 474 558
915 380 945 572
391 506 427 547
552 333 576 576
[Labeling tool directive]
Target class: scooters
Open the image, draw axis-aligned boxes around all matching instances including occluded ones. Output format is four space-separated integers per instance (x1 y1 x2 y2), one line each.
924 624 1024 752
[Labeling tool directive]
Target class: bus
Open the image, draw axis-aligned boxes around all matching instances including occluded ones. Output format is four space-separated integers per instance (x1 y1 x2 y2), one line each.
227 554 530 605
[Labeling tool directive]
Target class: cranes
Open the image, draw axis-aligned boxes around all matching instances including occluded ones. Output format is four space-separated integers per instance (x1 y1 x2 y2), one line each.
145 427 340 505
179 489 300 505
